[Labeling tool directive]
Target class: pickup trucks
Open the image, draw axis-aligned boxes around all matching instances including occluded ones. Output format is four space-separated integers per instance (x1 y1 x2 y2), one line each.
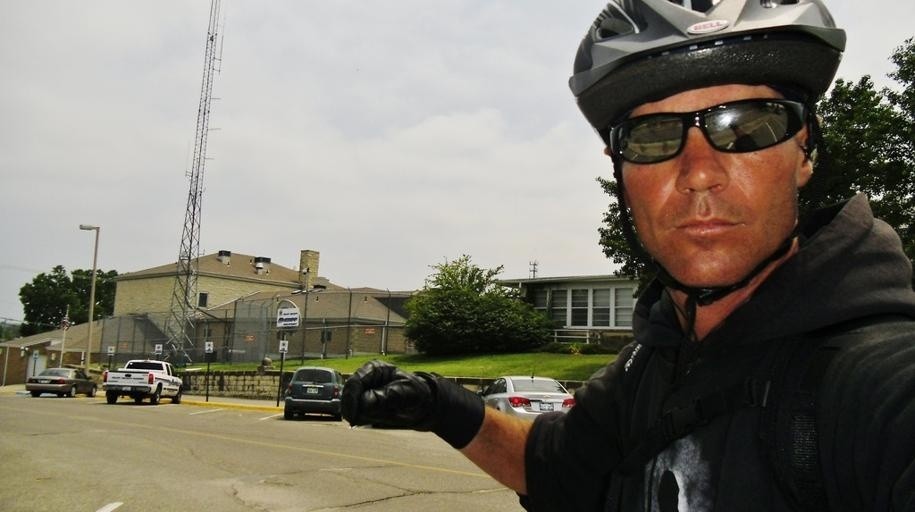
102 359 184 404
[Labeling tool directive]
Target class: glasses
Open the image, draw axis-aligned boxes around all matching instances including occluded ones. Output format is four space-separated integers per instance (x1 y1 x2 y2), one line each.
601 95 812 166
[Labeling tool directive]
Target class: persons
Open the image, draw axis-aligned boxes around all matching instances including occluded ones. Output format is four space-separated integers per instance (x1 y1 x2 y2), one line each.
340 0 915 510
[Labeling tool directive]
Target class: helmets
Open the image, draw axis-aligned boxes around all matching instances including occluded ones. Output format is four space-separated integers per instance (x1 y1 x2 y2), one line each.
566 0 848 144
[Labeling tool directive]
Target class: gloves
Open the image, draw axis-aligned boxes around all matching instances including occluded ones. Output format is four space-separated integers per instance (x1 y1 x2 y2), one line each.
339 359 487 453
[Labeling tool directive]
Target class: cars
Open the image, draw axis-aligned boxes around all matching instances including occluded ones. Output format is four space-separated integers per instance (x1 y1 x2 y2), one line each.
478 375 576 423
25 367 97 398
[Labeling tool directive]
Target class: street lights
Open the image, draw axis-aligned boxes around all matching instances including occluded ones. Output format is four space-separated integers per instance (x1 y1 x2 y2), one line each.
79 224 101 377
291 267 323 364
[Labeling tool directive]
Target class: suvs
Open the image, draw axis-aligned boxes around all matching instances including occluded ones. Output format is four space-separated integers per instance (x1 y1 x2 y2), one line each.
284 366 346 421
